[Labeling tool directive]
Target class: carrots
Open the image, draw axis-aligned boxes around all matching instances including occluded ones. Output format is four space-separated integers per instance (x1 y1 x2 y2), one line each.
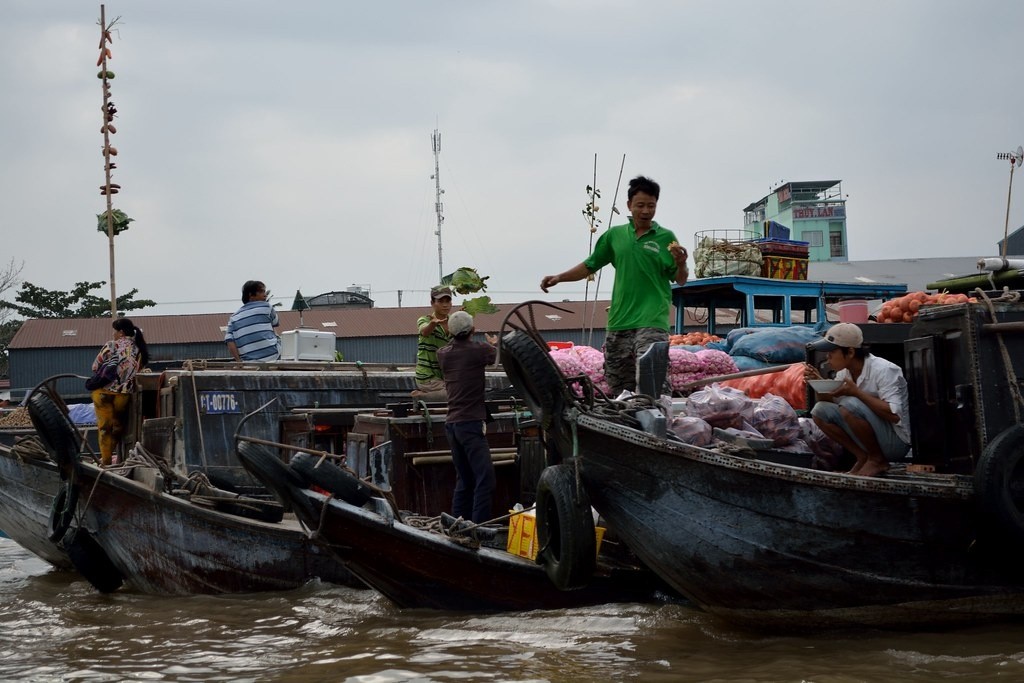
666 389 847 464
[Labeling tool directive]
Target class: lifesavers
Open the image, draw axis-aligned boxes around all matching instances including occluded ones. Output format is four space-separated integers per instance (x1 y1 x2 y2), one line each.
42 482 79 540
975 418 1024 562
535 466 598 591
25 391 77 464
290 448 370 505
62 528 121 593
235 439 300 498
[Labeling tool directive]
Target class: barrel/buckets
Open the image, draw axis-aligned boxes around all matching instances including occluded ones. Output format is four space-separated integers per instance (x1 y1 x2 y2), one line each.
838 300 868 323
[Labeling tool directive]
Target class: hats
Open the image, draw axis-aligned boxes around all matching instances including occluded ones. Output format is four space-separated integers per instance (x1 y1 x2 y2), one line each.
810 321 863 351
447 311 474 336
431 284 452 300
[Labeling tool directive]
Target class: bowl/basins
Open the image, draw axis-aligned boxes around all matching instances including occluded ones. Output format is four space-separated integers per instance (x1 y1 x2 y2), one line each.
807 380 845 393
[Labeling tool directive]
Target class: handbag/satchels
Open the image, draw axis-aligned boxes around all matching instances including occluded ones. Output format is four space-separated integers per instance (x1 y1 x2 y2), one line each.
86 347 119 391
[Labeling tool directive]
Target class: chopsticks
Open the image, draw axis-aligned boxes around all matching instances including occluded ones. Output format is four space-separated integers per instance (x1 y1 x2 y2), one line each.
802 363 825 380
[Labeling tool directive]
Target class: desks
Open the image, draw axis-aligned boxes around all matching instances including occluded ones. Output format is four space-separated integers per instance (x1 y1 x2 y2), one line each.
357 409 532 514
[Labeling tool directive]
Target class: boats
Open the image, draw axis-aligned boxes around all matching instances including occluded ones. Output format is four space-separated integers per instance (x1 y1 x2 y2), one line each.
0 230 661 609
492 147 1024 632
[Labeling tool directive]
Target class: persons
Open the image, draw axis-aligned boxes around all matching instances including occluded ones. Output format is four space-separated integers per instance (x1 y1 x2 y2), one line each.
224 281 282 367
90 318 142 466
539 176 689 399
414 285 498 527
803 322 912 477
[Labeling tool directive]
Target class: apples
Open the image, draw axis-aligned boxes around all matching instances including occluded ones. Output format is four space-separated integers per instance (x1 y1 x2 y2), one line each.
876 292 978 324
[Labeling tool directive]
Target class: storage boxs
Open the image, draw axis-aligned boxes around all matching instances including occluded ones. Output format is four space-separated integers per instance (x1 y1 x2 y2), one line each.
280 329 336 364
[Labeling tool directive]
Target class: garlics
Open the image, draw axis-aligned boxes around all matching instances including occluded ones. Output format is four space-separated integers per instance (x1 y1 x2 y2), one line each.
549 344 739 397
